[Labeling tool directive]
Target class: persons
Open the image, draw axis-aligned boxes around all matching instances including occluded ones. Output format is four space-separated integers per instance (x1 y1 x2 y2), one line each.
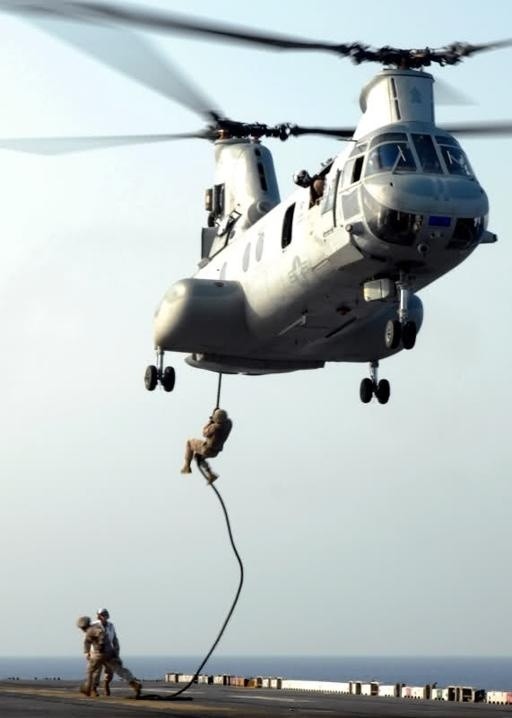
180 409 232 484
292 169 325 207
89 608 120 697
76 615 143 699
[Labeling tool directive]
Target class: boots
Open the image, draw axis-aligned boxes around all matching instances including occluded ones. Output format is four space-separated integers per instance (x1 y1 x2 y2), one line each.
80 684 99 696
182 464 192 474
209 471 216 485
104 680 110 696
129 680 141 698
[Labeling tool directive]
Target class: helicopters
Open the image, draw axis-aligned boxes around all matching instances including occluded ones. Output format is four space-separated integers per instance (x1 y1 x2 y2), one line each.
0 0 512 405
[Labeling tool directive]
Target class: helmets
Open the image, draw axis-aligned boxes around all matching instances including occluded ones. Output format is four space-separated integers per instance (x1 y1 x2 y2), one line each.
77 617 90 627
294 170 310 188
214 410 226 424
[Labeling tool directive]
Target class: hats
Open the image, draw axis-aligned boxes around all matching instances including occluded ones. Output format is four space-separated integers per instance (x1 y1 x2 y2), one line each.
98 608 110 618
